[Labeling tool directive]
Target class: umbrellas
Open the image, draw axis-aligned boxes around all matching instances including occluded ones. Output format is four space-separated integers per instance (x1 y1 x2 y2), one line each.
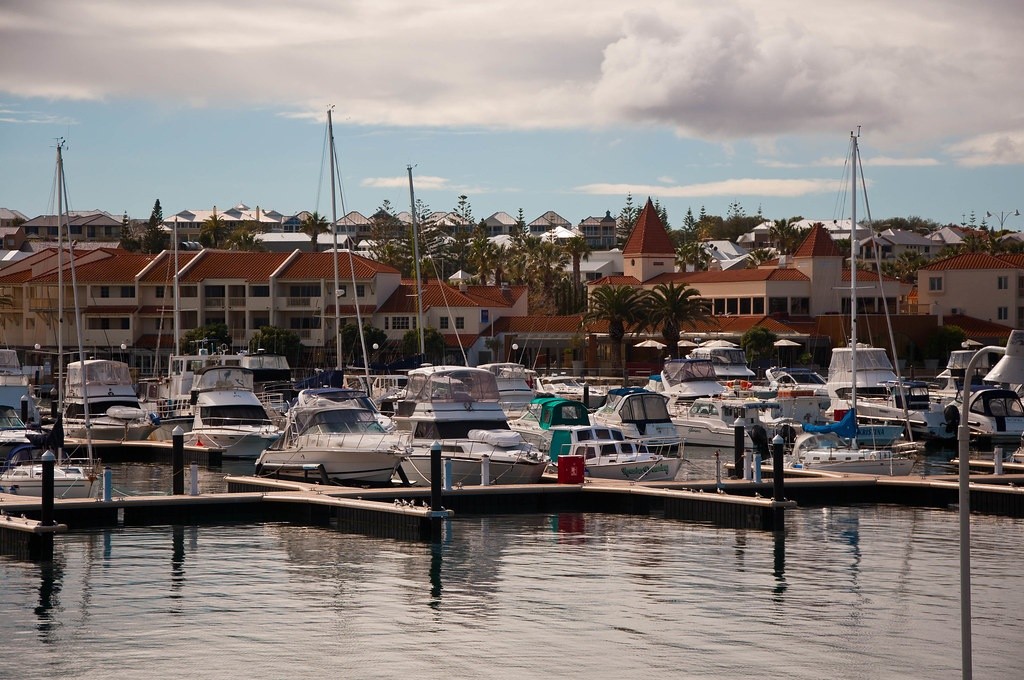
633 340 667 371
678 340 740 347
775 339 801 346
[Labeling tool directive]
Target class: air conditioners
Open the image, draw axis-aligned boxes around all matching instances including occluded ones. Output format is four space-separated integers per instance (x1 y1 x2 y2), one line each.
951 307 961 314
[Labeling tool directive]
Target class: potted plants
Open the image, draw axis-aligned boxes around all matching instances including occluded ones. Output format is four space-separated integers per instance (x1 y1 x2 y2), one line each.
767 216 804 269
680 243 703 272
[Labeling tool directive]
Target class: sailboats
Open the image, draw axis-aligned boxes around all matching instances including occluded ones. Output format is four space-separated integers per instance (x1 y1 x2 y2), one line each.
0 103 1024 500
